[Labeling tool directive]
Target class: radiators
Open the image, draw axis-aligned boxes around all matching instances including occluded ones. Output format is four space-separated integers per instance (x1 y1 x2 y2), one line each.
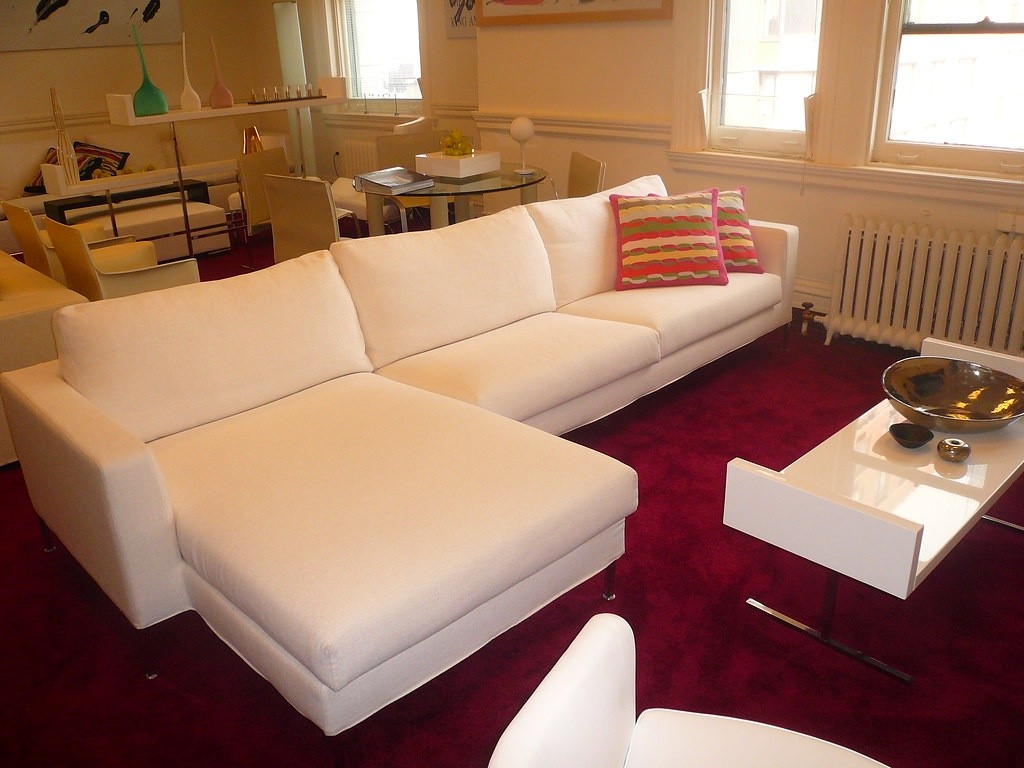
341 140 378 179
801 209 1024 359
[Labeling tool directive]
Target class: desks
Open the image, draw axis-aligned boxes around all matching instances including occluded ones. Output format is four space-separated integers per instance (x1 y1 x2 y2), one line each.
362 162 547 237
104 76 347 259
39 133 295 243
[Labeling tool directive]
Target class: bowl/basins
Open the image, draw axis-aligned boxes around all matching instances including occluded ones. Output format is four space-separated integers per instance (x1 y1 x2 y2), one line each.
881 356 1024 432
889 423 934 448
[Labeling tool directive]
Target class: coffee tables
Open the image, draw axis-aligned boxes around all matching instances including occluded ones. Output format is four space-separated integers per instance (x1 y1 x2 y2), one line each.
722 337 1024 682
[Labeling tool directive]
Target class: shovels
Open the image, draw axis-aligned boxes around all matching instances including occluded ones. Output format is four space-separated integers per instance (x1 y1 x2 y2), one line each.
179 32 201 112
209 34 234 110
131 23 170 117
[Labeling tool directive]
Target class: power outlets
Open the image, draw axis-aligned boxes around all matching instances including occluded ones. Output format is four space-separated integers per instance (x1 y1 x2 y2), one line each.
334 150 342 157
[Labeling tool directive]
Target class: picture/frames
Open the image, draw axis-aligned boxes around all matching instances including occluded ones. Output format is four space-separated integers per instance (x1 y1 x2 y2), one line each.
475 0 673 27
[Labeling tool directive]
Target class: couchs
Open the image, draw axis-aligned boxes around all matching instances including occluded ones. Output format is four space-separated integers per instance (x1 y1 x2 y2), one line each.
0 169 801 738
488 610 895 768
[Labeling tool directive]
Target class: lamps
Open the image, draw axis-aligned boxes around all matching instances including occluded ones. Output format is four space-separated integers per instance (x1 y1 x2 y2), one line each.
510 116 536 174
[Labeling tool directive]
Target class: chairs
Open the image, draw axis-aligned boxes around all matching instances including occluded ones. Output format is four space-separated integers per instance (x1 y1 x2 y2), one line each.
1 200 137 290
40 216 200 305
261 173 355 266
376 128 477 233
568 150 607 198
238 146 360 277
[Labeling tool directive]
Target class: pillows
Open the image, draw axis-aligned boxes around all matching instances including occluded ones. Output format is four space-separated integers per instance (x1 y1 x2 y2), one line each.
22 148 63 193
647 185 766 274
608 187 729 290
71 142 131 182
